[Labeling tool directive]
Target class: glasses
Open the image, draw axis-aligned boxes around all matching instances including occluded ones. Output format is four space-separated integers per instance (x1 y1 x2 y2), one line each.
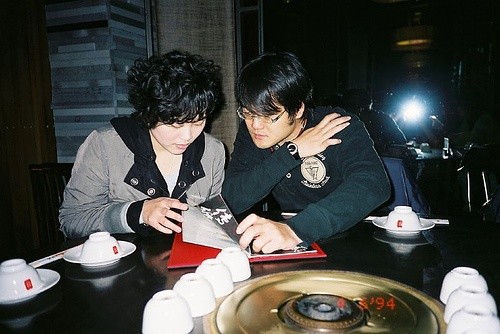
236 106 286 125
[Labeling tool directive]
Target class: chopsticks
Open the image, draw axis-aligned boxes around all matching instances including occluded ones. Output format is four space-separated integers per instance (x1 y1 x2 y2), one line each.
28 244 90 269
364 216 449 224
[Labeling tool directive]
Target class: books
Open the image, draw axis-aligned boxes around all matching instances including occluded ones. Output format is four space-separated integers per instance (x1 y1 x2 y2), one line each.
167 195 328 269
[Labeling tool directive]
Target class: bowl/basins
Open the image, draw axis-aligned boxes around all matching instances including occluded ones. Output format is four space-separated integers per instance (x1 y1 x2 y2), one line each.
0 259 44 300
79 232 122 261
386 206 422 229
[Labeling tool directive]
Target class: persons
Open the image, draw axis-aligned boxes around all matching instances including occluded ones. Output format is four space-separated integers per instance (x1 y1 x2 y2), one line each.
221 52 392 254
344 88 500 189
58 52 226 239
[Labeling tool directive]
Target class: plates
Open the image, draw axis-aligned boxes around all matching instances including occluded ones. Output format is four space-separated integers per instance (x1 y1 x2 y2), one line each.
372 216 433 237
0 268 60 305
63 240 137 267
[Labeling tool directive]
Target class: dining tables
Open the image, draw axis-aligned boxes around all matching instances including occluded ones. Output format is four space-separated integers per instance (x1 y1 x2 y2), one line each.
1 209 483 334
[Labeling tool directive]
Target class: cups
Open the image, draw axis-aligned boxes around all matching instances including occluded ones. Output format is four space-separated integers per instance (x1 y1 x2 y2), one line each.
446 304 500 334
443 284 497 323
141 290 195 334
213 246 252 282
173 272 218 318
440 266 488 305
194 258 235 298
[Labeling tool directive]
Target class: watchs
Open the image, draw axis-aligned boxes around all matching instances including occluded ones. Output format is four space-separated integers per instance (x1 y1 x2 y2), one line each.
284 141 301 162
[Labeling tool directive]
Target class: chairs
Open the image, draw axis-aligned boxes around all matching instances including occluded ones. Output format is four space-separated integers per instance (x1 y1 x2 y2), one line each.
382 158 429 221
23 162 74 256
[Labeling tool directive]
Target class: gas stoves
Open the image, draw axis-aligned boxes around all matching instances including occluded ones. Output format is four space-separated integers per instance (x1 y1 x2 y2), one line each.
204 270 446 334
